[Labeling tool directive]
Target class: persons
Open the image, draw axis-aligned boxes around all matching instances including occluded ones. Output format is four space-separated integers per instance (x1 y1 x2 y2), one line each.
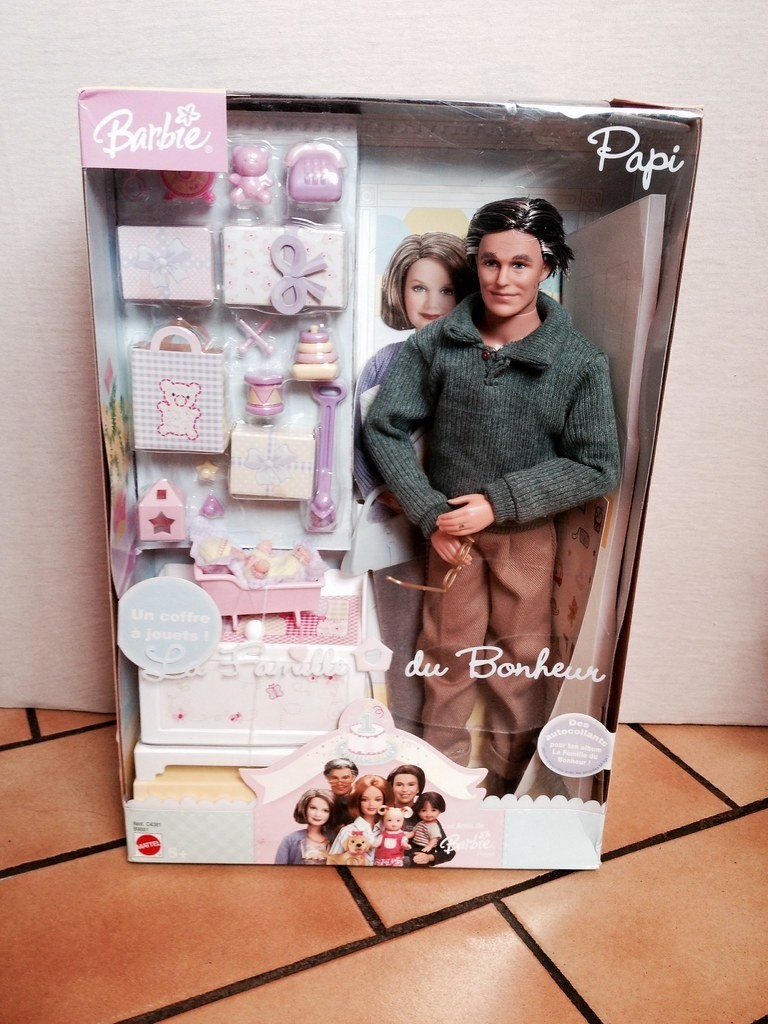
362 197 620 785
354 232 473 740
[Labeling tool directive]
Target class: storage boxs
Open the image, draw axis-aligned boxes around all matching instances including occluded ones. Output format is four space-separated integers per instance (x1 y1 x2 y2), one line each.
80 86 702 871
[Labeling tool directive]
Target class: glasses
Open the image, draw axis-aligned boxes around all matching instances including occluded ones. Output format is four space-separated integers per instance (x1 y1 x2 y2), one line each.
385 535 475 593
